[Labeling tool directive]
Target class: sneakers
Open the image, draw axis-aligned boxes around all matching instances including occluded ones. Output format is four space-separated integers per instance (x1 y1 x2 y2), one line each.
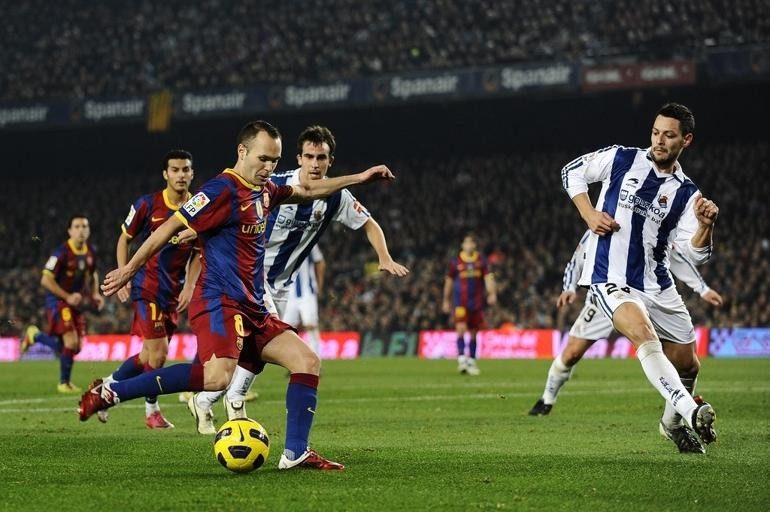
223 391 258 422
57 382 79 393
21 325 40 353
144 410 174 429
178 390 217 434
528 399 553 415
278 446 344 471
77 377 109 425
458 362 480 376
659 395 716 454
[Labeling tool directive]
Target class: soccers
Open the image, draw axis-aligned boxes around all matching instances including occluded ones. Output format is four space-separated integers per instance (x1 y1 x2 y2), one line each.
213 417 270 473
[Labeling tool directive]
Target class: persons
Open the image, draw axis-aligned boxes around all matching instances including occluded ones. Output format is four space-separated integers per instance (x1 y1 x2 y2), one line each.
77 120 395 470
528 230 723 418
441 233 496 377
178 125 410 435
178 352 258 403
284 243 326 380
561 103 719 453
21 215 103 394
88 150 203 431
1 1 769 335
